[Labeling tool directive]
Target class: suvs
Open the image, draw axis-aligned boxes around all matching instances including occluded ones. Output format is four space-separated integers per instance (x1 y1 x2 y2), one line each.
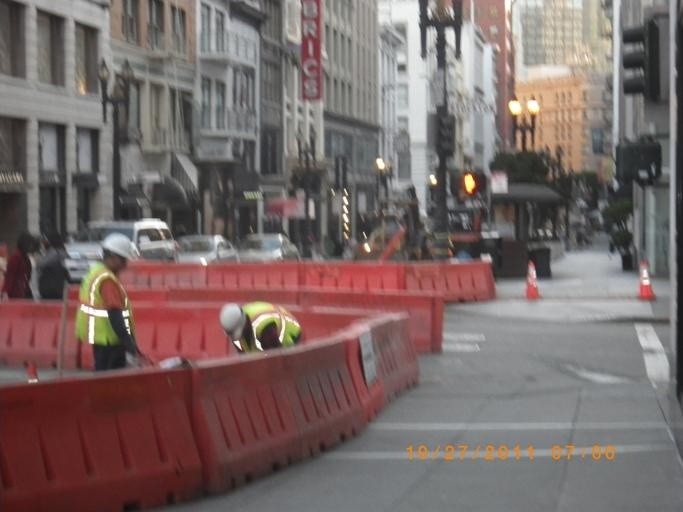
59 215 179 284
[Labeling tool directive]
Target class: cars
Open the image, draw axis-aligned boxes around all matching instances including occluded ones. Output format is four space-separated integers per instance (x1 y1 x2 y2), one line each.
362 225 402 258
236 231 302 263
173 232 242 265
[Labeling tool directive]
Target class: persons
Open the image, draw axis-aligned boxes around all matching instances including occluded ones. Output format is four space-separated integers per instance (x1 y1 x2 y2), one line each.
74 230 156 372
1 226 42 303
219 297 305 357
36 235 72 299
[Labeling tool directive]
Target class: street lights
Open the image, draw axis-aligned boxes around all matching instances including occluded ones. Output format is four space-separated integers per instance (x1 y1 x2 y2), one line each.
94 55 137 218
416 0 465 258
544 140 562 239
372 154 396 201
505 92 540 152
292 123 319 244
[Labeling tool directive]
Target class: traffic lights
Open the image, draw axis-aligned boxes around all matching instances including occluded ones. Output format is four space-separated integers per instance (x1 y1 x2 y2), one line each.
460 169 481 197
622 19 660 103
433 111 457 160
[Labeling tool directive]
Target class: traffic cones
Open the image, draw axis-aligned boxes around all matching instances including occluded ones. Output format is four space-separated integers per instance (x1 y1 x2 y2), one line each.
635 262 660 301
24 357 40 383
524 259 544 301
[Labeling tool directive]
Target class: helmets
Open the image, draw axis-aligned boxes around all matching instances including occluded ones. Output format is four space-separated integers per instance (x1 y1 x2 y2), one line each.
218 302 247 342
101 232 140 263
